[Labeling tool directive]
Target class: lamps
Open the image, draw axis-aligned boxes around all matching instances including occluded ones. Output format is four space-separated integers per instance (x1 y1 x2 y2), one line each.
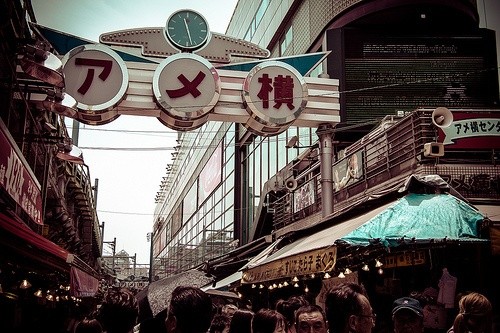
285 178 297 192
429 107 455 130
20 278 83 304
252 254 388 292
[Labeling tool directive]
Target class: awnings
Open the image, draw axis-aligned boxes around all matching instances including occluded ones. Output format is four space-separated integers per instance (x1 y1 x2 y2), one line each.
237 193 493 284
145 255 215 319
200 270 243 293
0 212 101 298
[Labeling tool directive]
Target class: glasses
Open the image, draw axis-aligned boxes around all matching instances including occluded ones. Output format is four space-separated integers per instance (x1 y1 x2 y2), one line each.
350 313 376 323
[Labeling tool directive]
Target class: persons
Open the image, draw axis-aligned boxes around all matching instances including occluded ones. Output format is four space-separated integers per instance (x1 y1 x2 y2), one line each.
55 282 500 333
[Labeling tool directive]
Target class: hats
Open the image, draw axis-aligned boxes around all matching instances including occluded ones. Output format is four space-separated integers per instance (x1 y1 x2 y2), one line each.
392 297 421 316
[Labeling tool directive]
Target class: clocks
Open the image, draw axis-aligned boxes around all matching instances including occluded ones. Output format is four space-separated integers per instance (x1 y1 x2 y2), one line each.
166 9 210 48
